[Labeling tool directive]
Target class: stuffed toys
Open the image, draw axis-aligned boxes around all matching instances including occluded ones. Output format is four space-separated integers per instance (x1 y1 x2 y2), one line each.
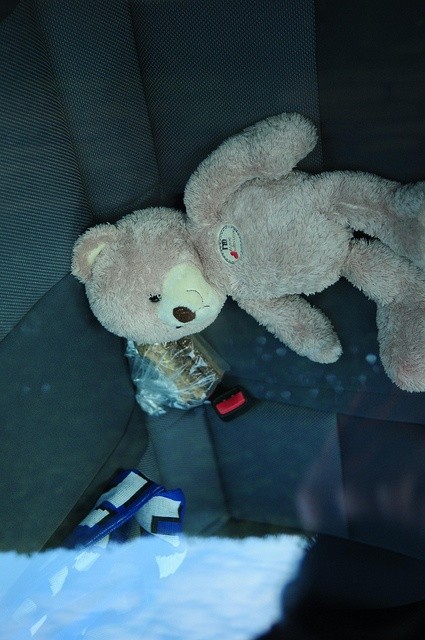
70 112 425 393
